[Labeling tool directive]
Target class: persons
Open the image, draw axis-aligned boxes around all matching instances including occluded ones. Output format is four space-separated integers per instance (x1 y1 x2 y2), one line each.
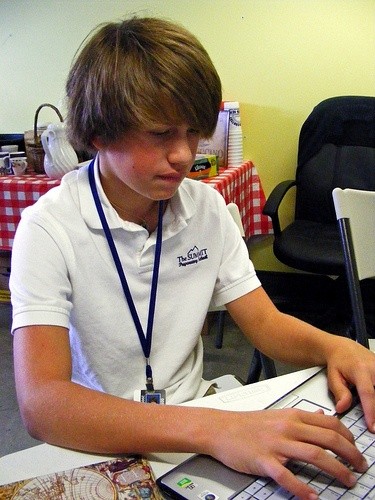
9 16 375 500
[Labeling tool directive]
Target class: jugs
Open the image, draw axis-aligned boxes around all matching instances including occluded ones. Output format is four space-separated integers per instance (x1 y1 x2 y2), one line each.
41 121 79 179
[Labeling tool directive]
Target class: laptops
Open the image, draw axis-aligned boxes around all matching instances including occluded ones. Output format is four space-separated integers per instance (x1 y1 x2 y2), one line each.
154 363 375 500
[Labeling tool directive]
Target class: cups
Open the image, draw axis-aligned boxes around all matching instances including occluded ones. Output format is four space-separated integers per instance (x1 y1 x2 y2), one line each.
223 99 243 166
0 145 28 176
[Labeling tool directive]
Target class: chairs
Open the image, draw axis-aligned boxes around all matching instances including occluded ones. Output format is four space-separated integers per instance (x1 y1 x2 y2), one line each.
261 95 375 273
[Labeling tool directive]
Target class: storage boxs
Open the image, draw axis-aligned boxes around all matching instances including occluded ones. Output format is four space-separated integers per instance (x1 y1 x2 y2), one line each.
188 154 221 180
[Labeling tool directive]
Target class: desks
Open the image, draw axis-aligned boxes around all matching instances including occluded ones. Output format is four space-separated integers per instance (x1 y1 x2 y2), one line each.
0 156 271 350
0 363 375 500
